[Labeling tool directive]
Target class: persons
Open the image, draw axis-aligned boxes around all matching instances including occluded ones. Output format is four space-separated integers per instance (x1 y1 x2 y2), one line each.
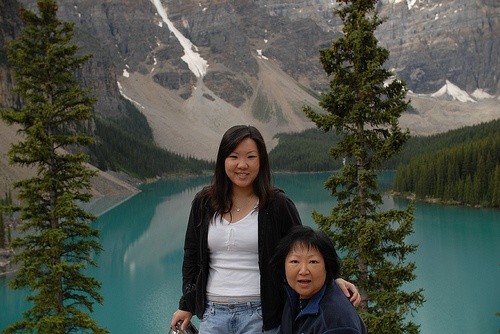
274 226 367 334
170 125 362 334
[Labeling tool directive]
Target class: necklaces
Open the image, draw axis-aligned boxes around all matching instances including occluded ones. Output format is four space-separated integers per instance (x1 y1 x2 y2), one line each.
235 207 241 212
